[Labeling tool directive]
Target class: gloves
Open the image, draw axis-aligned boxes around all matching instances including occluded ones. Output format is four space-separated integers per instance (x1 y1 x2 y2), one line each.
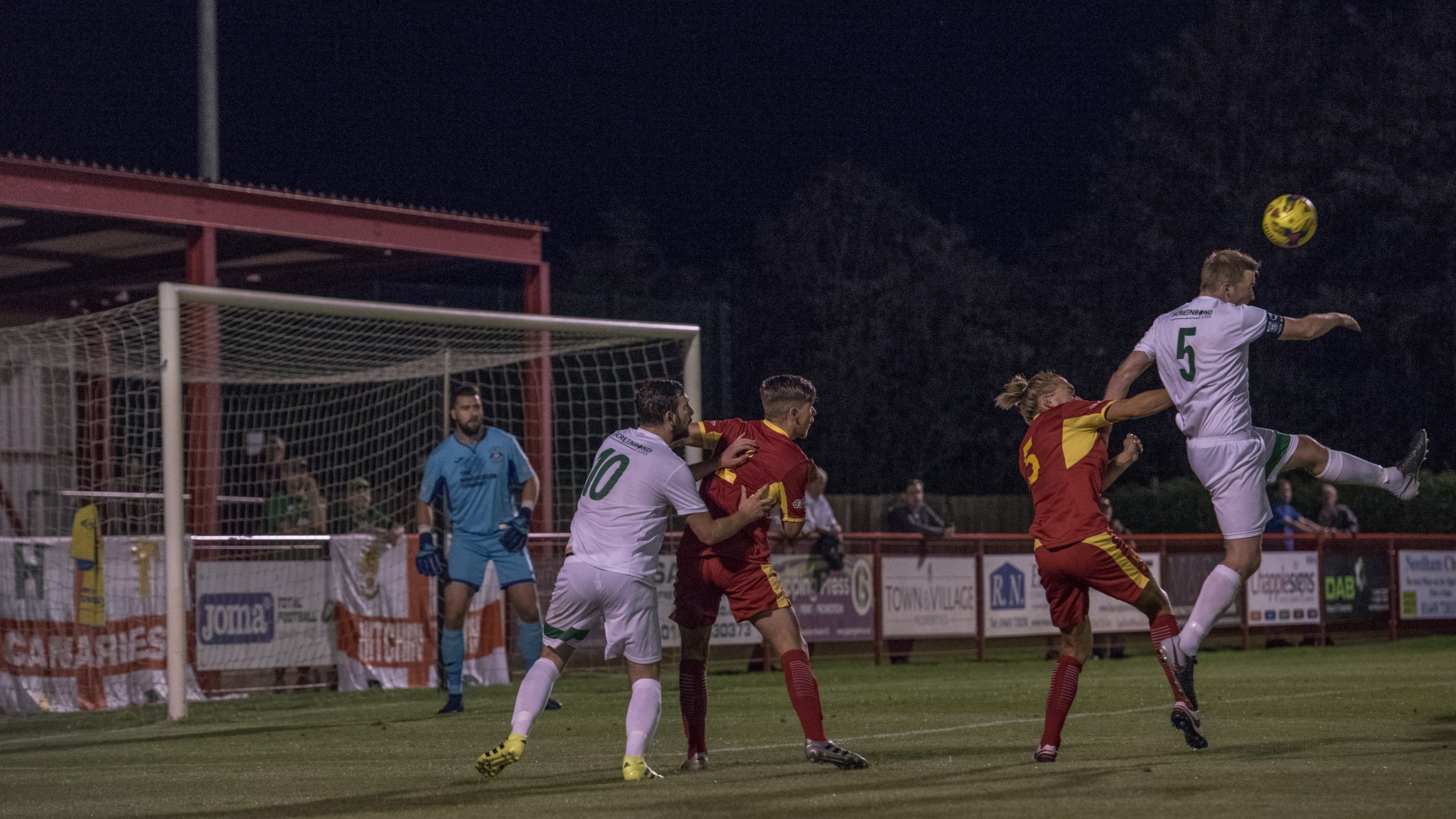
415 533 442 577
498 505 532 553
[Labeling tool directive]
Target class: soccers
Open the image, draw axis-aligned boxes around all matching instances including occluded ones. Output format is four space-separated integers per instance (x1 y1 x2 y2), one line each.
1261 193 1318 249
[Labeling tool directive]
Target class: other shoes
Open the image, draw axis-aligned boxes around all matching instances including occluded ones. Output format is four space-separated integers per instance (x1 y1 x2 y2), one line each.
273 680 286 694
296 677 311 693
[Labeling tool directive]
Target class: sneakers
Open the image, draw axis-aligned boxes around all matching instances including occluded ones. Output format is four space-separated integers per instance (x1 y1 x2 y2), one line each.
1391 429 1428 501
805 738 870 771
622 755 665 781
545 697 561 711
1170 701 1209 749
436 698 464 715
681 753 709 770
1159 635 1199 712
474 734 528 776
1033 742 1058 762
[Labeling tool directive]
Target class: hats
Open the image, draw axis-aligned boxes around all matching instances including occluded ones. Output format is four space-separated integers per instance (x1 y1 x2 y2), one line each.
348 476 369 490
283 456 307 471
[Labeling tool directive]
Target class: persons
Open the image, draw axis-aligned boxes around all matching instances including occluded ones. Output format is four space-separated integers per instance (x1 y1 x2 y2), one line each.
236 436 404 543
418 385 561 719
1101 250 1429 706
668 372 1362 767
472 380 781 781
